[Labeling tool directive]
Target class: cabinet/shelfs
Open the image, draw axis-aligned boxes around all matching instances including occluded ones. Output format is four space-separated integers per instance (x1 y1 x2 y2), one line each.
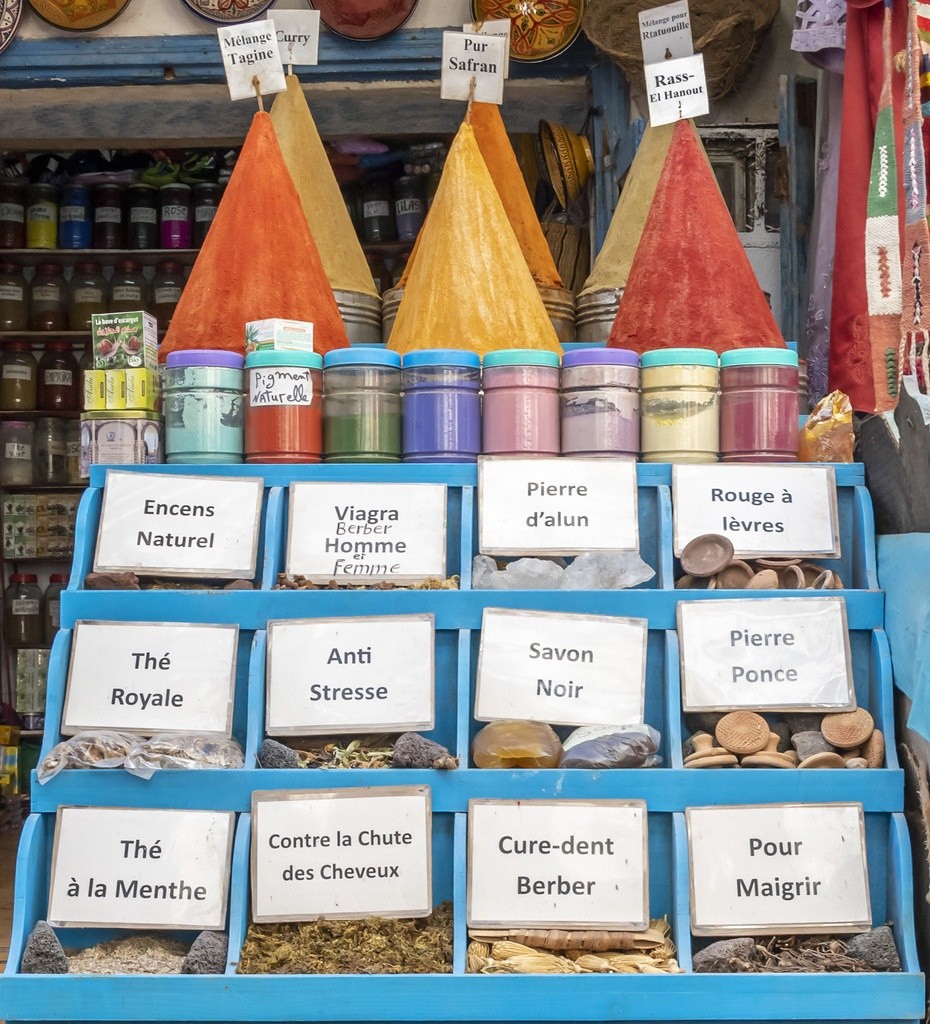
0 175 414 804
0 460 926 1024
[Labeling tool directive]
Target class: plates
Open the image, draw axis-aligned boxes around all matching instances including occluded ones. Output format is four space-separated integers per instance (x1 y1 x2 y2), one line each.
178 0 278 25
0 0 23 53
469 0 585 64
308 0 418 41
28 0 133 31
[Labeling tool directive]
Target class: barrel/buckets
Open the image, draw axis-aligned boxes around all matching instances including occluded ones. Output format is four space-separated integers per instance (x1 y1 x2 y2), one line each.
330 286 627 350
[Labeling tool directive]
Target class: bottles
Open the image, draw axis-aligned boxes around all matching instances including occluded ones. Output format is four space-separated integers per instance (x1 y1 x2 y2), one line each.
6 573 71 645
403 348 483 463
561 348 639 458
0 178 224 413
161 349 246 465
720 348 802 462
0 417 92 485
341 171 442 292
322 347 402 463
482 349 560 458
245 350 322 464
639 347 720 462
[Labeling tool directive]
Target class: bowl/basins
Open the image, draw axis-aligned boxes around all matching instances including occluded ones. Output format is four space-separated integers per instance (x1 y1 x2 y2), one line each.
538 118 598 212
